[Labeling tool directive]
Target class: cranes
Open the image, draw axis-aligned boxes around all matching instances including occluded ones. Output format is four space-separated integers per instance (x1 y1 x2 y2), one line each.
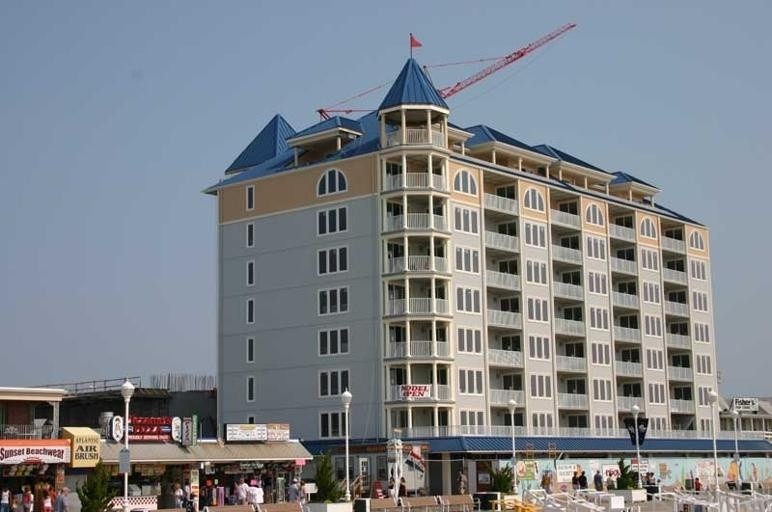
316 21 577 122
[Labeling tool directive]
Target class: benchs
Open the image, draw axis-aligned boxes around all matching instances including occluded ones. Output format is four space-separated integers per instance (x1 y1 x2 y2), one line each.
260 501 307 512
371 498 399 512
204 504 258 512
439 493 475 512
401 495 439 511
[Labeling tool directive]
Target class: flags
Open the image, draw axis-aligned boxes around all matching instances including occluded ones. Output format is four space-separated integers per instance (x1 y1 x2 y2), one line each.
405 451 426 473
622 417 636 445
637 417 649 445
411 36 424 48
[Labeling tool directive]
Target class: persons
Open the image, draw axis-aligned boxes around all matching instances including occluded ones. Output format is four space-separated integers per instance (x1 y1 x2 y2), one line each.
577 470 588 489
693 477 703 495
593 469 603 491
398 476 408 497
175 475 308 511
644 472 649 485
0 480 71 511
649 471 656 485
454 470 468 495
606 473 614 489
540 471 552 493
387 477 396 497
570 471 579 490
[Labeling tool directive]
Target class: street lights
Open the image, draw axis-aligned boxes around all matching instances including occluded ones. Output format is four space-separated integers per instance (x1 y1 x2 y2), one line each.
731 409 742 488
507 399 519 494
121 377 135 512
341 387 353 502
707 391 720 492
631 404 642 489
